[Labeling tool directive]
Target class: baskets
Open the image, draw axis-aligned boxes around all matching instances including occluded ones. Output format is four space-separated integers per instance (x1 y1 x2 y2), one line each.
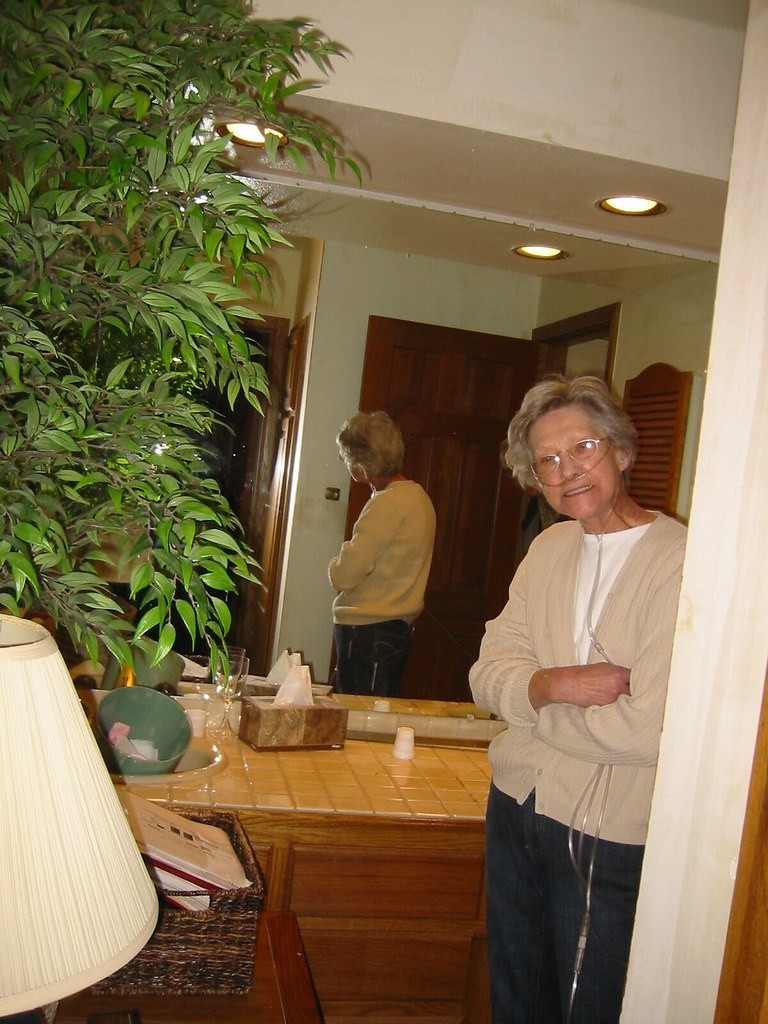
88 807 264 996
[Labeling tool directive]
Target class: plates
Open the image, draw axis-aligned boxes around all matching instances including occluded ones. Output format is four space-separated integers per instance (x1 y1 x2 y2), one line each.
88 721 228 784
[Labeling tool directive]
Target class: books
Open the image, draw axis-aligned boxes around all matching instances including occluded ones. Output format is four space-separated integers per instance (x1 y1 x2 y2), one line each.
114 790 251 911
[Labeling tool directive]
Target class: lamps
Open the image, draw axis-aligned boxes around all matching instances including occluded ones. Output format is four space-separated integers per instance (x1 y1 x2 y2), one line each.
0 612 159 1020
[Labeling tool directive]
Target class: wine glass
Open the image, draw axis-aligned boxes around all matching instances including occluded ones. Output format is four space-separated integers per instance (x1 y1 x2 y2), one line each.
212 654 249 738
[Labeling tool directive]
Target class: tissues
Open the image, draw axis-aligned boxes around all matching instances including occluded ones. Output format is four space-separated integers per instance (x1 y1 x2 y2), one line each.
235 650 333 696
238 665 349 753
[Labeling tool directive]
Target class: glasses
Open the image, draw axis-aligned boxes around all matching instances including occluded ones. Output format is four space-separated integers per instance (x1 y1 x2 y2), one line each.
528 436 611 477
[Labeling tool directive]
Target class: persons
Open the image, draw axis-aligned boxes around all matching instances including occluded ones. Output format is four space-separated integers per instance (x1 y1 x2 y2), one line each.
469 375 689 1024
329 411 436 699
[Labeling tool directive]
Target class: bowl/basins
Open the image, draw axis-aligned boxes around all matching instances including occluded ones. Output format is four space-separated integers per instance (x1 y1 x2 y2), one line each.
93 686 193 772
134 640 185 690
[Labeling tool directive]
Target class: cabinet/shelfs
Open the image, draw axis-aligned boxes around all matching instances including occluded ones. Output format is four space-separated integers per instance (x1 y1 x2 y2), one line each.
110 738 492 1024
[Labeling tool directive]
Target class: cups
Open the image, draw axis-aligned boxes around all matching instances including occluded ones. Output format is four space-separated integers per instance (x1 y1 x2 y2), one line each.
391 727 415 759
374 700 390 713
216 646 246 701
185 709 208 738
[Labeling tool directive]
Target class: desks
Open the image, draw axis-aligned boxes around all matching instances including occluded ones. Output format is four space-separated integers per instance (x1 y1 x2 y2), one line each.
52 909 323 1024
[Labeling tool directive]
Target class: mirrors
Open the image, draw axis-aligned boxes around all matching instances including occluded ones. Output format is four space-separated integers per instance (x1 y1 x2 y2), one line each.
42 179 718 749
